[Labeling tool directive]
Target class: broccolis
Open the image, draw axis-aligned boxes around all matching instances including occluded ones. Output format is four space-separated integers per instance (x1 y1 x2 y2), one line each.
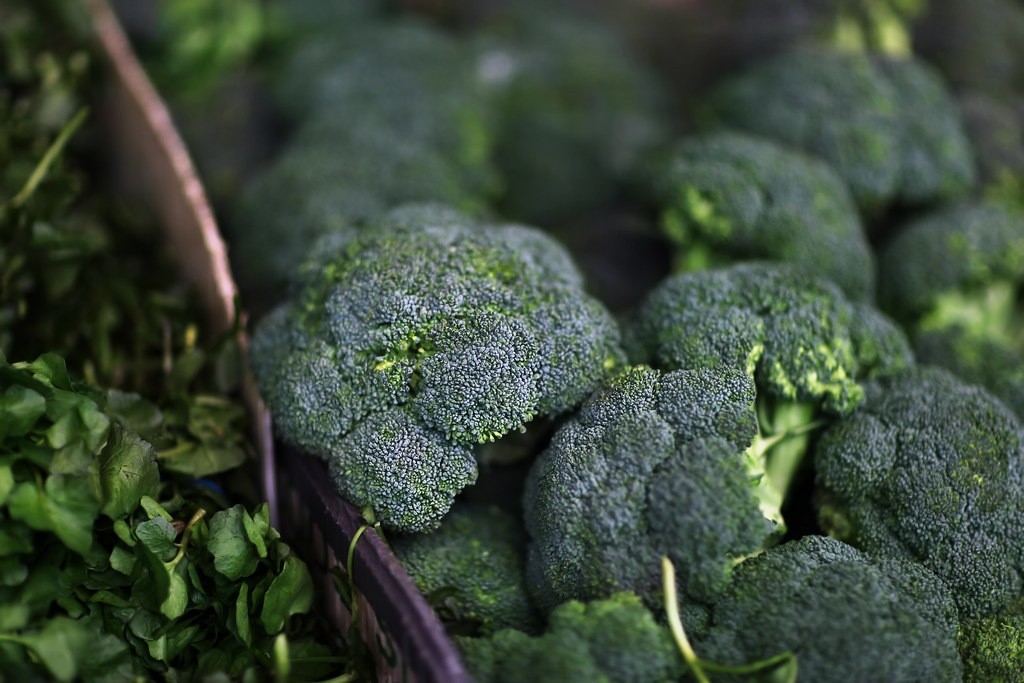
126 0 1024 683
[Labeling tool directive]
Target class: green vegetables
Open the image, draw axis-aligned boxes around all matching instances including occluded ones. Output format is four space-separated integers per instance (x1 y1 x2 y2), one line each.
0 0 376 683
